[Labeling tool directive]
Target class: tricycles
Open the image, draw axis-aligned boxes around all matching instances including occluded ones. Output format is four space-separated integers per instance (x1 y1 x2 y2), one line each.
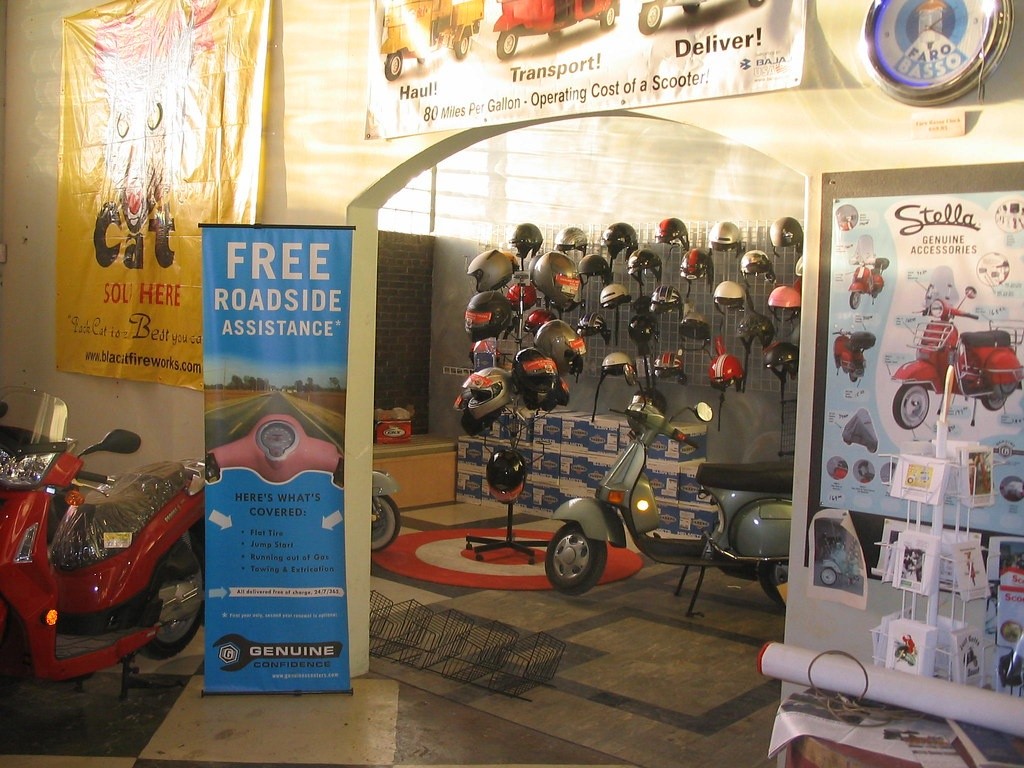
381 0 767 83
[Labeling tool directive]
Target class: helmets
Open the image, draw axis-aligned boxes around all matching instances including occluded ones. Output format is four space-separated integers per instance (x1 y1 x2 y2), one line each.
509 223 543 258
512 319 586 411
709 250 803 390
554 227 588 251
680 248 707 280
601 223 638 260
523 309 556 336
486 448 528 504
469 340 501 365
506 283 537 311
708 222 740 251
770 217 803 247
656 218 687 243
453 366 513 420
529 252 581 309
625 248 662 275
576 254 712 415
465 291 512 342
501 252 520 271
466 250 513 293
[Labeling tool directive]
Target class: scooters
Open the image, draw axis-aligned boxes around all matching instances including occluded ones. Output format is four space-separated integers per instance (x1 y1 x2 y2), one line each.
892 282 1024 431
545 361 793 618
0 386 205 701
833 325 877 383
848 253 889 310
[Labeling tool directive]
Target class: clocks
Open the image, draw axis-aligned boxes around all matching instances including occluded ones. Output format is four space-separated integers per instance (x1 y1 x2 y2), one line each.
858 0 1015 106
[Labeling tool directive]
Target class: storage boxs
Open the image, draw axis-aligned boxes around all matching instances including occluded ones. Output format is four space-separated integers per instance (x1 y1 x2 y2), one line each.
561 415 596 454
482 440 508 476
588 415 627 457
532 483 599 518
648 422 708 462
482 477 532 513
534 411 589 451
587 453 619 490
645 461 679 505
646 502 718 540
559 452 589 490
374 421 482 474
680 459 720 509
506 408 568 446
618 422 636 453
509 447 532 483
478 410 514 442
456 472 481 506
532 450 561 489
